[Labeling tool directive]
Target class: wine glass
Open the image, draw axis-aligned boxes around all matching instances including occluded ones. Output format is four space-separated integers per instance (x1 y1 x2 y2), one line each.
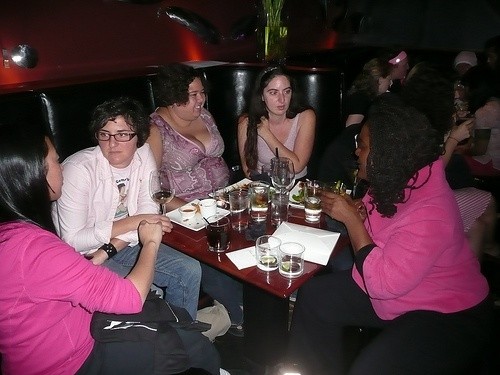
270 157 296 220
148 169 175 217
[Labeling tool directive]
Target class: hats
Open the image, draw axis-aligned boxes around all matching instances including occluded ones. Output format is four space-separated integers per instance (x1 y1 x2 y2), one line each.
454 51 477 67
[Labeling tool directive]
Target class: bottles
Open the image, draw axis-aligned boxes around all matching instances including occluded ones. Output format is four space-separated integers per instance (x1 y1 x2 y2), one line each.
453 78 474 146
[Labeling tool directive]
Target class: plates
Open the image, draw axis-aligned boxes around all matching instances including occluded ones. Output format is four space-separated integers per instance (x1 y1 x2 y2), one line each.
165 199 230 231
209 178 279 210
288 180 352 209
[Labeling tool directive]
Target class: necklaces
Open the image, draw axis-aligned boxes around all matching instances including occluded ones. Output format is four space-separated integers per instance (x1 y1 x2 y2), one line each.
168 109 193 129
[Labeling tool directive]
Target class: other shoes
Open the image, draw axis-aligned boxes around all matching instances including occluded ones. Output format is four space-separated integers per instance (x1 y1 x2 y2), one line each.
212 294 249 337
482 243 500 259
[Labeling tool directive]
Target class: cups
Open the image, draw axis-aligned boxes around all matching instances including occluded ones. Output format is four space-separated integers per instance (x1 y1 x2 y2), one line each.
177 206 197 218
199 199 218 218
204 215 231 252
255 235 281 271
228 191 249 231
467 127 491 155
277 240 306 277
304 180 323 222
246 182 271 222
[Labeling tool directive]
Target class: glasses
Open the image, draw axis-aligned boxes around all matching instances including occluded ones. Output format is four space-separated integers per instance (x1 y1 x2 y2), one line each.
95 132 138 142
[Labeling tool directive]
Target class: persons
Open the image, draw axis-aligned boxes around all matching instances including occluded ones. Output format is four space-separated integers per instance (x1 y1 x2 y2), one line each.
287 102 495 374
0 121 222 375
329 47 500 261
237 64 317 189
139 62 246 340
50 96 201 322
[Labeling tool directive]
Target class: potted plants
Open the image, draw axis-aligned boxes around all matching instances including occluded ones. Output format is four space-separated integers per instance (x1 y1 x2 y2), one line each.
256 0 290 67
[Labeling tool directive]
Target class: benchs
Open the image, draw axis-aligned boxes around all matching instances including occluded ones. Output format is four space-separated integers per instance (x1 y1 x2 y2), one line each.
0 66 358 230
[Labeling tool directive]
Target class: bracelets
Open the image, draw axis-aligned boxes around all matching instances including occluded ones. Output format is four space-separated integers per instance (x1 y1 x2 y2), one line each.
448 136 459 142
98 243 118 259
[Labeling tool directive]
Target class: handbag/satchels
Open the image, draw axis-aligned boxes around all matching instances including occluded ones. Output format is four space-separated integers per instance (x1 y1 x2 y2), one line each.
90 289 213 341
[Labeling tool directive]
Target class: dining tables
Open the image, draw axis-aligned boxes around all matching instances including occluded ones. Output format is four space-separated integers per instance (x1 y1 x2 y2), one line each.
161 203 351 337
